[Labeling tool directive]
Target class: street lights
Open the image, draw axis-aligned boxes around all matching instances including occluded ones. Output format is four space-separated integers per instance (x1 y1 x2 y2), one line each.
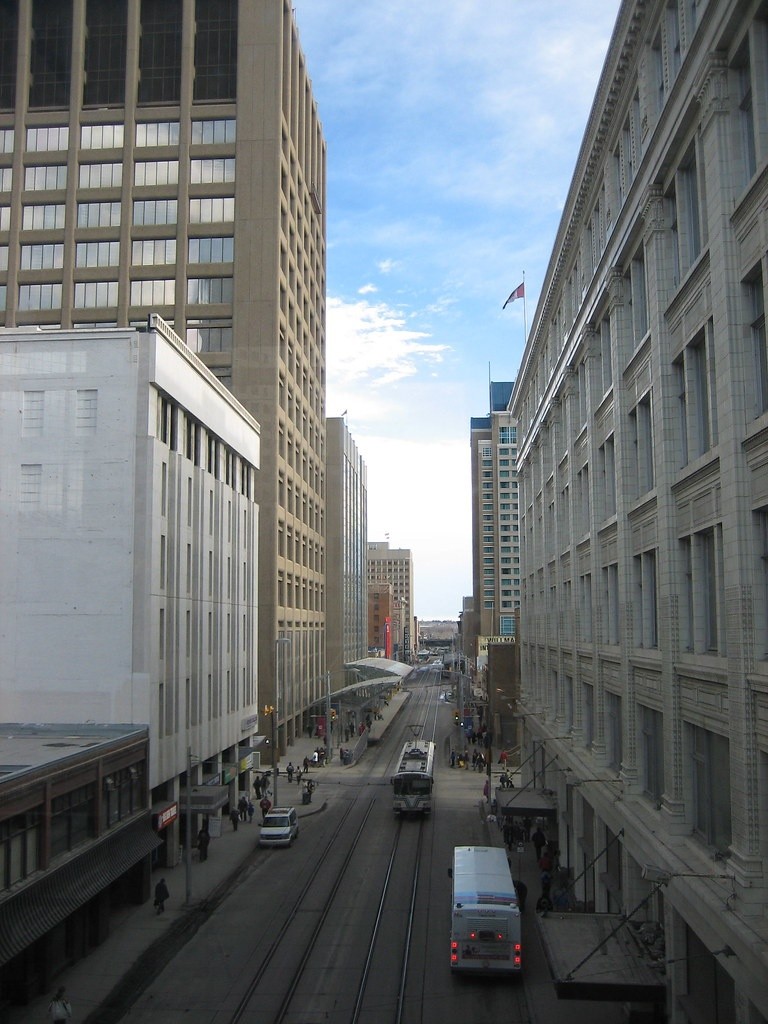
433 668 465 759
325 668 361 761
272 637 291 806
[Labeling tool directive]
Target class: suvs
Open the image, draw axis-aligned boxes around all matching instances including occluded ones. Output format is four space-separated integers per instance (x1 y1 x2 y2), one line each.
257 805 299 848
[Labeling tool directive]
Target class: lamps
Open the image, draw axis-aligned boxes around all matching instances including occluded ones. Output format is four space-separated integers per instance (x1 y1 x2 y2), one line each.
564 775 624 787
512 712 543 720
500 695 528 702
530 735 573 744
640 863 733 886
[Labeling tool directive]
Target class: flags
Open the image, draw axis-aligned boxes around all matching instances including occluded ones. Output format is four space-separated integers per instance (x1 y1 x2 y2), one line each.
503 282 524 309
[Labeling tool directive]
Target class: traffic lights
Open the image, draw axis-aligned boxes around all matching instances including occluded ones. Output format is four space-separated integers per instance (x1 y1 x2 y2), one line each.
454 709 460 722
460 722 464 727
264 705 274 717
329 710 336 721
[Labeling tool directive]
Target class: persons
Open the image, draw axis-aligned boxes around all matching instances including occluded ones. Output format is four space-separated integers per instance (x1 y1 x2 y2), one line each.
238 797 248 821
313 747 327 767
340 747 343 760
253 776 261 799
286 763 294 783
229 807 239 831
155 878 169 915
359 715 372 736
501 815 568 912
451 702 514 803
197 830 210 860
345 723 354 741
303 757 309 773
247 802 254 822
294 766 302 785
261 775 272 798
48 986 72 1024
260 796 270 817
302 779 314 802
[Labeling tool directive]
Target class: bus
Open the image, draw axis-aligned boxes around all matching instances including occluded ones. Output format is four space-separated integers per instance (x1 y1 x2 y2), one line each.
445 845 524 981
391 724 437 816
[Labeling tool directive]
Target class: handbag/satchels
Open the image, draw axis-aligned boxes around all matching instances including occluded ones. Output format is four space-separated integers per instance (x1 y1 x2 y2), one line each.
497 759 501 765
460 761 464 767
154 899 159 906
313 755 316 759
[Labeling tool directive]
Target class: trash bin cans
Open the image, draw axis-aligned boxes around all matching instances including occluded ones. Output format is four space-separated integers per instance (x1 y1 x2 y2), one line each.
343 752 351 764
302 792 310 805
